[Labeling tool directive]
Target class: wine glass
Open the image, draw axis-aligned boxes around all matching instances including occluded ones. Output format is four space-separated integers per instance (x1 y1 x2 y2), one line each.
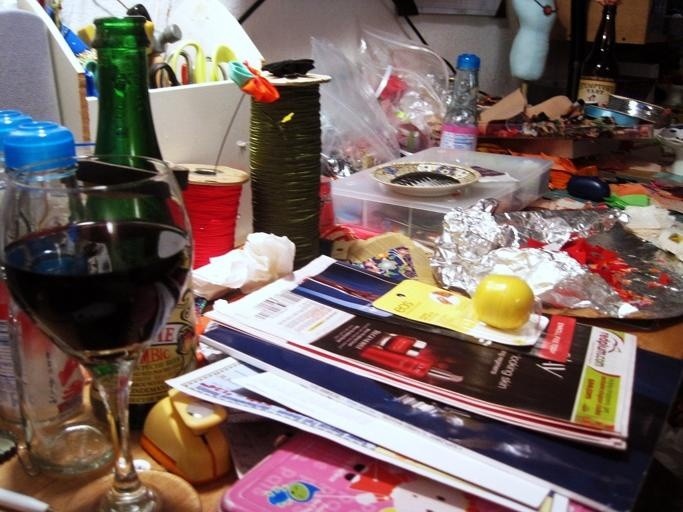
2 155 199 512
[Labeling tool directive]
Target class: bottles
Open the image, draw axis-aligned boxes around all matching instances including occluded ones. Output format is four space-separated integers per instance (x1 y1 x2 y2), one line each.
89 18 194 428
2 110 86 433
575 4 619 105
439 52 479 153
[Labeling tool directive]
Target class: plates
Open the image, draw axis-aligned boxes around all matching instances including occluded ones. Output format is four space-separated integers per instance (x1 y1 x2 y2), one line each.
376 161 484 197
